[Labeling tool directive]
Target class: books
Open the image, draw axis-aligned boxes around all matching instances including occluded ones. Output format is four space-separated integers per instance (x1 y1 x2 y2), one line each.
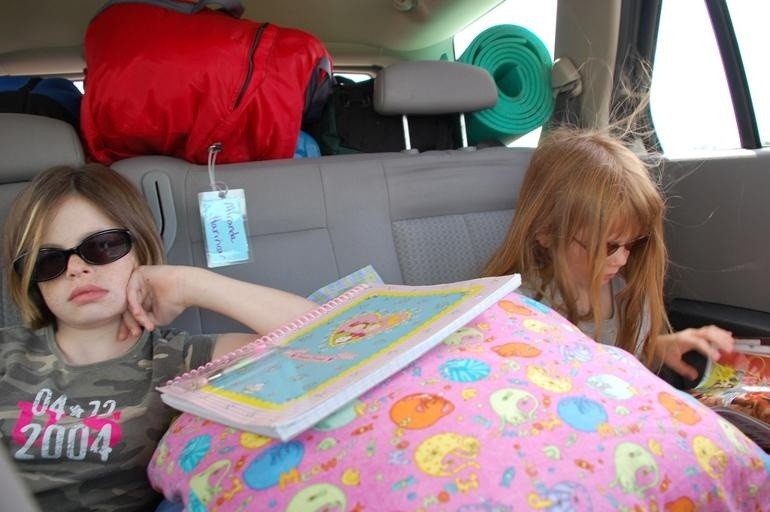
683 336 769 452
158 268 525 442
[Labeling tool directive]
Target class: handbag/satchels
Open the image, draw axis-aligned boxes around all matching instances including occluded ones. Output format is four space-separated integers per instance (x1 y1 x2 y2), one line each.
0 76 82 133
80 0 331 167
302 79 464 159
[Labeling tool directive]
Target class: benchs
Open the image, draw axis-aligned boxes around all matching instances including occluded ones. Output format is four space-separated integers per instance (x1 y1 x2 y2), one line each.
0 58 537 335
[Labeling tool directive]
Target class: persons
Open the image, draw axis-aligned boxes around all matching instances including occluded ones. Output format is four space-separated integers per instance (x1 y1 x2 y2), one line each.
0 161 321 511
476 124 735 390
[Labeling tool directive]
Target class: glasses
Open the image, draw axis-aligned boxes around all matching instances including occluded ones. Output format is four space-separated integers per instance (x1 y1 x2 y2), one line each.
12 229 137 282
572 236 649 258
